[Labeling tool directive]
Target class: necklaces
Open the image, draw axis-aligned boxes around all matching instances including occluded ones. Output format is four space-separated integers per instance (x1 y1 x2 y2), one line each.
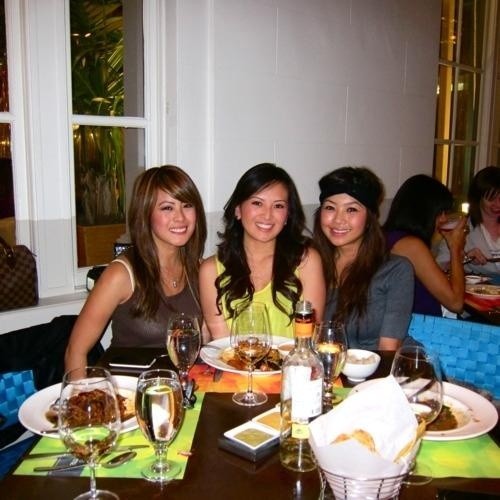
154 259 186 292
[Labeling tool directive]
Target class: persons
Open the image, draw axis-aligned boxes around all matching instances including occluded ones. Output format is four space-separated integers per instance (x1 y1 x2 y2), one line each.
198 162 328 350
311 167 417 362
380 173 469 318
433 165 499 282
59 163 208 385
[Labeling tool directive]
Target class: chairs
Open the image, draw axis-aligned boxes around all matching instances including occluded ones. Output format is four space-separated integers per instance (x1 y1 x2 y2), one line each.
0 315 106 391
408 313 500 399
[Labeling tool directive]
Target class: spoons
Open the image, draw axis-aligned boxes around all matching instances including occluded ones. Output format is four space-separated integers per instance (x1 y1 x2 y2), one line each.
33 452 137 475
197 362 213 380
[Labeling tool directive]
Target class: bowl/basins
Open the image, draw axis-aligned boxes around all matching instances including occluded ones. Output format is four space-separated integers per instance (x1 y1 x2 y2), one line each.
342 347 382 384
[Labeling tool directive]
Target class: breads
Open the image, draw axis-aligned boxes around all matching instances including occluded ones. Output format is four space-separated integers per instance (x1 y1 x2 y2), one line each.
330 430 375 452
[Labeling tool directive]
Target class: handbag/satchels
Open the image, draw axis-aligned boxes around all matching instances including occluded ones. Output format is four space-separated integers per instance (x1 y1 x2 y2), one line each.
0 237 38 313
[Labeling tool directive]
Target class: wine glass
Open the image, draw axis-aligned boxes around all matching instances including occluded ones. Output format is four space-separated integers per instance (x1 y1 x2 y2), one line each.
389 344 445 488
58 366 127 499
438 213 474 266
164 311 200 409
134 370 187 485
230 300 274 406
309 319 347 412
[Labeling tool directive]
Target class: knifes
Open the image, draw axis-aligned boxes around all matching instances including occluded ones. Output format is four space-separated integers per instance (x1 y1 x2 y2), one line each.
212 370 225 383
20 445 151 459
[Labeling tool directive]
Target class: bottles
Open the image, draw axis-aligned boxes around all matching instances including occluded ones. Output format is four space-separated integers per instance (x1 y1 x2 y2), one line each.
281 300 324 474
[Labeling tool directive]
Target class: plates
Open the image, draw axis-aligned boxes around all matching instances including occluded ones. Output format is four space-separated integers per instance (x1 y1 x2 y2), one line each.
19 374 139 441
465 273 482 286
464 284 500 302
374 375 499 445
221 402 290 455
197 335 295 378
